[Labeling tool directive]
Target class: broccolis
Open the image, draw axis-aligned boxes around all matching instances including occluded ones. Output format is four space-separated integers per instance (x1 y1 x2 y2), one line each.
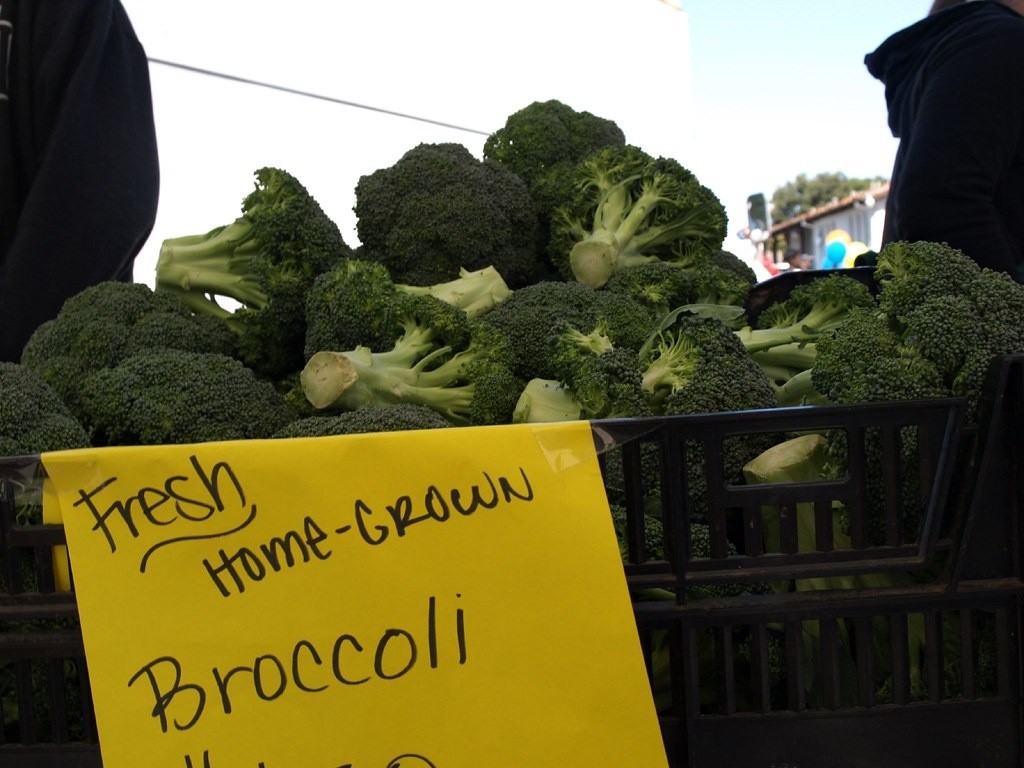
0 102 1023 741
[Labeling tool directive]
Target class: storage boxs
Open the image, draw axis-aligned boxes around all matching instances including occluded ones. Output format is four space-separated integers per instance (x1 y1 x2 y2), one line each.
0 356 1023 768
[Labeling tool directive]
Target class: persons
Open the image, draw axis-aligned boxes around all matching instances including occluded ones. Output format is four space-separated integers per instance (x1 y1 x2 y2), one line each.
799 252 813 271
781 248 802 274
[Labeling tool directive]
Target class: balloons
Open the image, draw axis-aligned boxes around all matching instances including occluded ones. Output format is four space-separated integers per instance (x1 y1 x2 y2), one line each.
737 227 771 244
821 228 866 268
